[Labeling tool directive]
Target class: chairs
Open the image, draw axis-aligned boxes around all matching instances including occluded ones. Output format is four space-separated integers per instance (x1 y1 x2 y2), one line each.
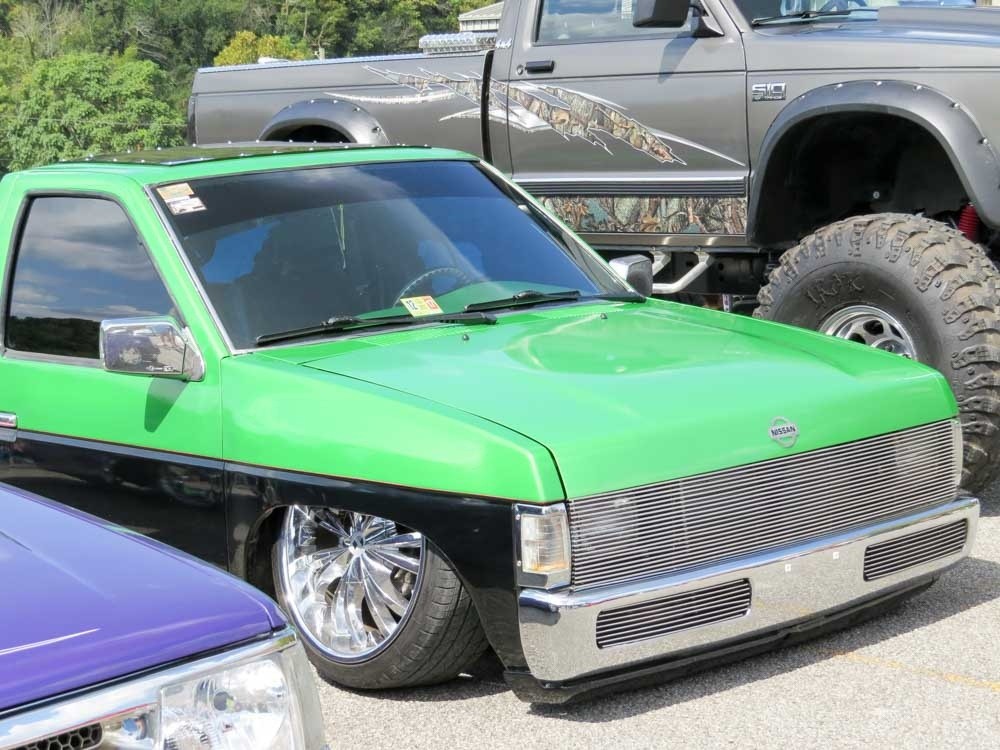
550 0 633 37
117 211 385 348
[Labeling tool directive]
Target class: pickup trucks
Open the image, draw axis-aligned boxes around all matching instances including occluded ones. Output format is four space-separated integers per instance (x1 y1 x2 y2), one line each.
183 1 1000 501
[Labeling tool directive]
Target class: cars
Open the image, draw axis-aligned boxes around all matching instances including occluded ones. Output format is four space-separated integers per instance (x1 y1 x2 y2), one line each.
0 479 327 750
0 139 983 710
